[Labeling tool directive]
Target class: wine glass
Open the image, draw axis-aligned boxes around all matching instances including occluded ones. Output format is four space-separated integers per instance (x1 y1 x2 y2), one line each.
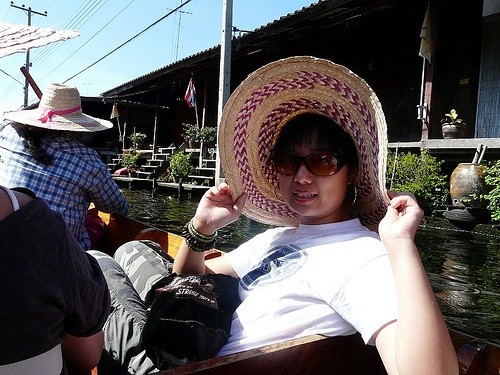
208 147 215 160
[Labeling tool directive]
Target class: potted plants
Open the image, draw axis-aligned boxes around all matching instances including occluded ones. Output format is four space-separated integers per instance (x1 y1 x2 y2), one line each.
118 147 142 178
440 109 467 139
169 151 195 183
127 132 147 149
181 123 218 148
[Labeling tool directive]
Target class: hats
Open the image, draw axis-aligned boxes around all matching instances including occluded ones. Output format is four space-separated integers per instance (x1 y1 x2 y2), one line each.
219 56 392 232
4 83 113 132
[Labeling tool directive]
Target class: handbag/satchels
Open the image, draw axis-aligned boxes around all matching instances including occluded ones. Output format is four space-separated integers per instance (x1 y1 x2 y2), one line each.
139 273 242 371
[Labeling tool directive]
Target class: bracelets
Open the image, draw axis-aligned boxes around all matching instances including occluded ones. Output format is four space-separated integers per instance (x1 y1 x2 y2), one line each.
183 218 216 253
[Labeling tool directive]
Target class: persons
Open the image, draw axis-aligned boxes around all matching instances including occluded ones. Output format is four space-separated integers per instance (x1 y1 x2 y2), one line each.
86 55 460 375
0 184 112 375
0 82 129 251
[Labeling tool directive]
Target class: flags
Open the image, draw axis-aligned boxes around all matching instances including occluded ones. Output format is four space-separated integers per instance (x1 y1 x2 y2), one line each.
419 3 434 63
110 98 118 119
185 76 196 108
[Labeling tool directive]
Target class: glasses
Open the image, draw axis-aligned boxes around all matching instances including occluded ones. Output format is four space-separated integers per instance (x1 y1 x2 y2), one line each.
272 150 349 176
